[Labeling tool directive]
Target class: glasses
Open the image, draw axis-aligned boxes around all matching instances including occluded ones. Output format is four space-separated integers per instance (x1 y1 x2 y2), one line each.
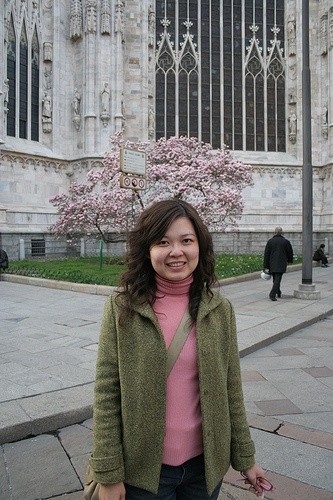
237 470 275 492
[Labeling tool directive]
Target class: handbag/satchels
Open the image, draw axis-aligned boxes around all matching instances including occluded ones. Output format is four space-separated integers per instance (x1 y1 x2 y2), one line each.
83 448 100 499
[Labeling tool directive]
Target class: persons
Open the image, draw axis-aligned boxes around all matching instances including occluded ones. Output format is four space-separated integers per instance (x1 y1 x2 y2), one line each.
288 110 297 134
88 199 266 500
313 244 331 268
322 102 328 122
72 87 81 115
42 90 52 120
148 105 155 129
100 82 111 112
2 78 10 108
263 226 294 301
